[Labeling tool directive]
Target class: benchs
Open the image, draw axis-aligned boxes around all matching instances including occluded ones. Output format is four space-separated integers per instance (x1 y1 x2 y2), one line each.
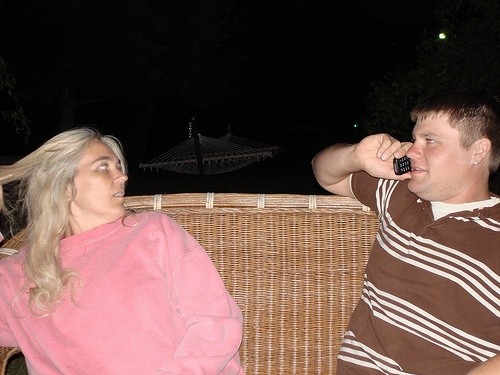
0 193 379 375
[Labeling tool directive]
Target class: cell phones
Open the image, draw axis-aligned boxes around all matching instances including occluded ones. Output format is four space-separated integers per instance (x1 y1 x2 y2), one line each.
393 155 411 175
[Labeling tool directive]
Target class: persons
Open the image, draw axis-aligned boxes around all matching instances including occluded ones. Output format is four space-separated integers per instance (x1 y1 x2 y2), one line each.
310 87 500 374
0 127 249 375
